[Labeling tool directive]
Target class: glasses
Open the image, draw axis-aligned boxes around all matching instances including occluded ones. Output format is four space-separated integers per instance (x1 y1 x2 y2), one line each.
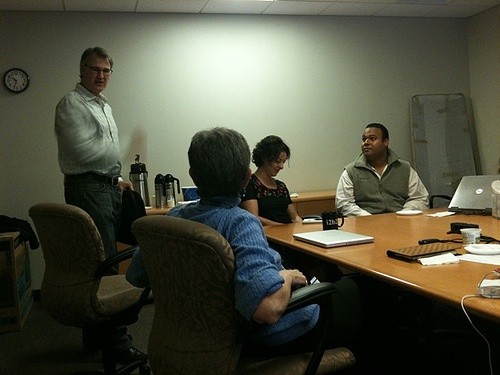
84 64 113 75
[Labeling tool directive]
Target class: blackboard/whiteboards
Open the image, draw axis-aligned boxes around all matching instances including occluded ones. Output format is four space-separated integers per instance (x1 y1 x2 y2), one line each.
410 93 476 208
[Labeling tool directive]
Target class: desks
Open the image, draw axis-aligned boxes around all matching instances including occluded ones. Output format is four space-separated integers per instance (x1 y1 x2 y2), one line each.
290 189 336 217
263 208 500 325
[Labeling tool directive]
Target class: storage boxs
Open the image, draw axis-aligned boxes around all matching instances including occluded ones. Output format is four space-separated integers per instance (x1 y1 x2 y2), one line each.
0 231 34 332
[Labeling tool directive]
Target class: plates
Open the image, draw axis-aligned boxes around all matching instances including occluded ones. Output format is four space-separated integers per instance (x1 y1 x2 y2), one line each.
463 244 500 255
396 210 422 215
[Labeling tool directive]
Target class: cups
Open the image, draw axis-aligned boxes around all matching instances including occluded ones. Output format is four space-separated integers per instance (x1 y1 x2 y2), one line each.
322 211 344 230
460 228 482 246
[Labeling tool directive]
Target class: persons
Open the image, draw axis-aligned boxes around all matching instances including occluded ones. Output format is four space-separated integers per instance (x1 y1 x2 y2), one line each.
336 123 430 217
54 47 149 365
124 129 363 359
242 135 344 285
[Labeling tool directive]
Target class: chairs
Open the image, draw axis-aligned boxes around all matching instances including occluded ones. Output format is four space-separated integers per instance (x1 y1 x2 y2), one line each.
132 215 357 375
28 203 154 375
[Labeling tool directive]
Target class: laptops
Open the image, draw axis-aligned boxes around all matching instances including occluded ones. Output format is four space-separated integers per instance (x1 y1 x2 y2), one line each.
291 228 376 248
447 175 500 215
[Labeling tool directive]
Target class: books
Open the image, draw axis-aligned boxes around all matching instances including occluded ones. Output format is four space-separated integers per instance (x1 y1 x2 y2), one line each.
387 243 467 263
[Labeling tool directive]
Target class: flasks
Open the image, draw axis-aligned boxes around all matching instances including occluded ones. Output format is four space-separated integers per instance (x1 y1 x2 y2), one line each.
129 164 150 207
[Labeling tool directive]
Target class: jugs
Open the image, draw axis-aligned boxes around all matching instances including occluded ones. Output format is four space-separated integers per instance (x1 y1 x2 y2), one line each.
155 173 181 209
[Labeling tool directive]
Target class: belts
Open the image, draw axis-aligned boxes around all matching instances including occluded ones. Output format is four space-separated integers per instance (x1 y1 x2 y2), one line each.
64 171 122 186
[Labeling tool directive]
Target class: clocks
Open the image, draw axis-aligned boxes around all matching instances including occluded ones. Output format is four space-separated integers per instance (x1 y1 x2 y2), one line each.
4 68 29 93
